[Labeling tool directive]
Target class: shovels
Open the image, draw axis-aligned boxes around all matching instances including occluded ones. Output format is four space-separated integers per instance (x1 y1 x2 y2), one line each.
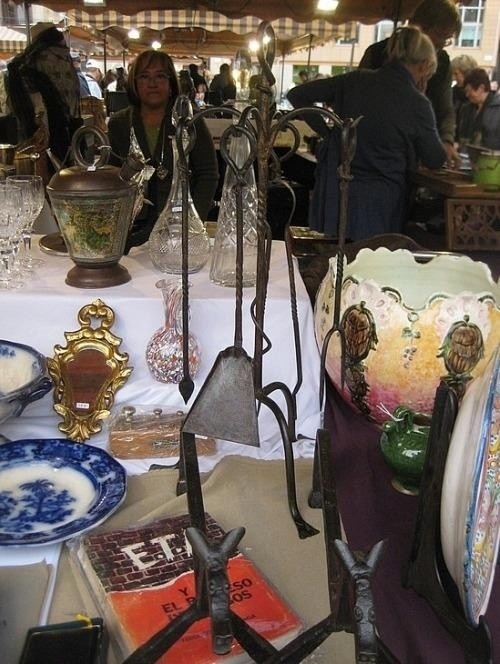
179 126 259 448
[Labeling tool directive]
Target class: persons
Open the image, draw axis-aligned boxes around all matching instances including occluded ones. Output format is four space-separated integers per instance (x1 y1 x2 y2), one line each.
209 62 237 118
107 50 220 256
298 70 320 83
285 25 463 242
3 21 84 175
0 50 208 115
450 52 479 138
357 0 463 173
456 67 499 152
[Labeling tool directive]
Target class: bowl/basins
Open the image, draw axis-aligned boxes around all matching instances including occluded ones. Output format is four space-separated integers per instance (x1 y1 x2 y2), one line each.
0 338 46 425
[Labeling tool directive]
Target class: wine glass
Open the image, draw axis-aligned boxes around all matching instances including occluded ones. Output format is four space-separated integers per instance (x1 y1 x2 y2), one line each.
1 174 43 292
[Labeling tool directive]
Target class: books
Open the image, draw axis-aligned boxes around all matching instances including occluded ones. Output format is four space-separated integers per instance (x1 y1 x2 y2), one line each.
75 510 305 664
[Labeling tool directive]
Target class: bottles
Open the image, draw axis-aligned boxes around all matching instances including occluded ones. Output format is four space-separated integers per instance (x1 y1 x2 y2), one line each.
144 278 200 382
381 407 431 495
150 95 266 288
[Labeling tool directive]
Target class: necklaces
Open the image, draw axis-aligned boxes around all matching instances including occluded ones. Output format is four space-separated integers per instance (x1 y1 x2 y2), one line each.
156 126 168 181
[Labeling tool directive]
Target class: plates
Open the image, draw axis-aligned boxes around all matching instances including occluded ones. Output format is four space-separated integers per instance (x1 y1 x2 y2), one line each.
0 439 126 548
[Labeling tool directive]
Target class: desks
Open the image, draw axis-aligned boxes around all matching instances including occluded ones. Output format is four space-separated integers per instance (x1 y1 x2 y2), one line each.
0 234 310 422
401 167 499 283
204 117 317 148
